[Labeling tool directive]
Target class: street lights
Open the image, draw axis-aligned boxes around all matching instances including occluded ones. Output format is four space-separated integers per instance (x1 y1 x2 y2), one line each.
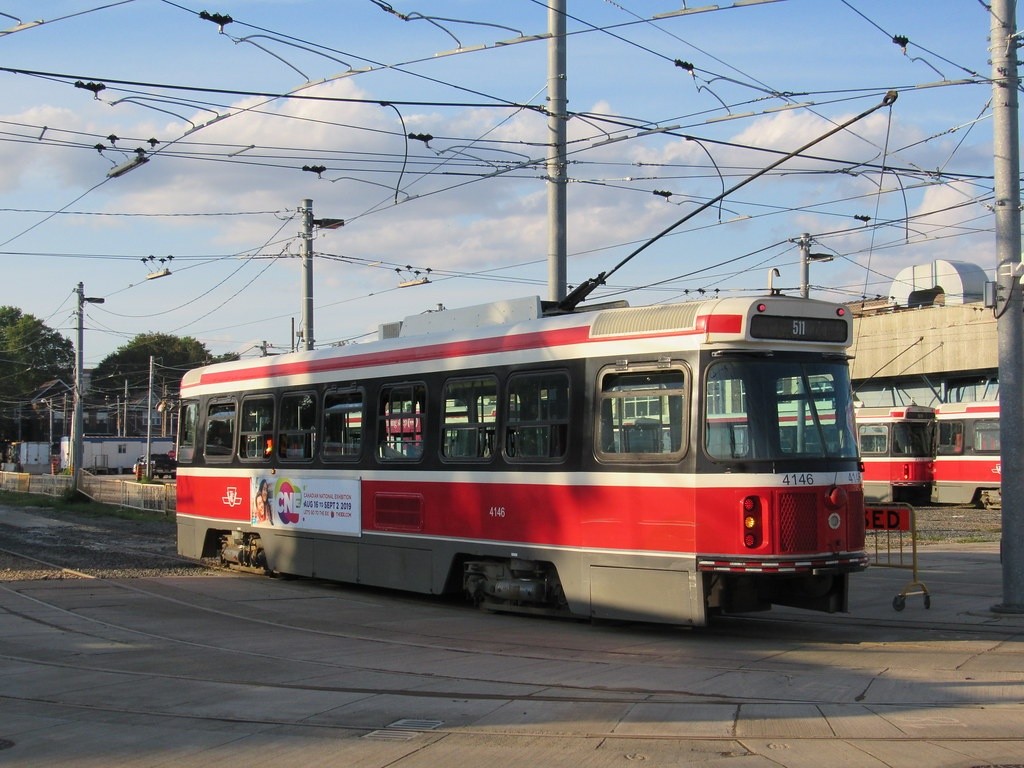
299 219 345 350
41 398 52 455
70 297 105 488
796 253 836 452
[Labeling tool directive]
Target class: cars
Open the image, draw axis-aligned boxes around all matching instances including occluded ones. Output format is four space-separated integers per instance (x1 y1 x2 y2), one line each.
133 453 177 480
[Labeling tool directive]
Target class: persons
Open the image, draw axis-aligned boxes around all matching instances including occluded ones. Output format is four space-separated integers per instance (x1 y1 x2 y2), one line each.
257 479 274 525
253 492 272 526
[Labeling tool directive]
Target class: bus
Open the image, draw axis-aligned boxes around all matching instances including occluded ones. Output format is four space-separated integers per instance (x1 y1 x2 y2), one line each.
344 405 521 458
928 400 1000 509
614 403 938 488
176 296 871 629
